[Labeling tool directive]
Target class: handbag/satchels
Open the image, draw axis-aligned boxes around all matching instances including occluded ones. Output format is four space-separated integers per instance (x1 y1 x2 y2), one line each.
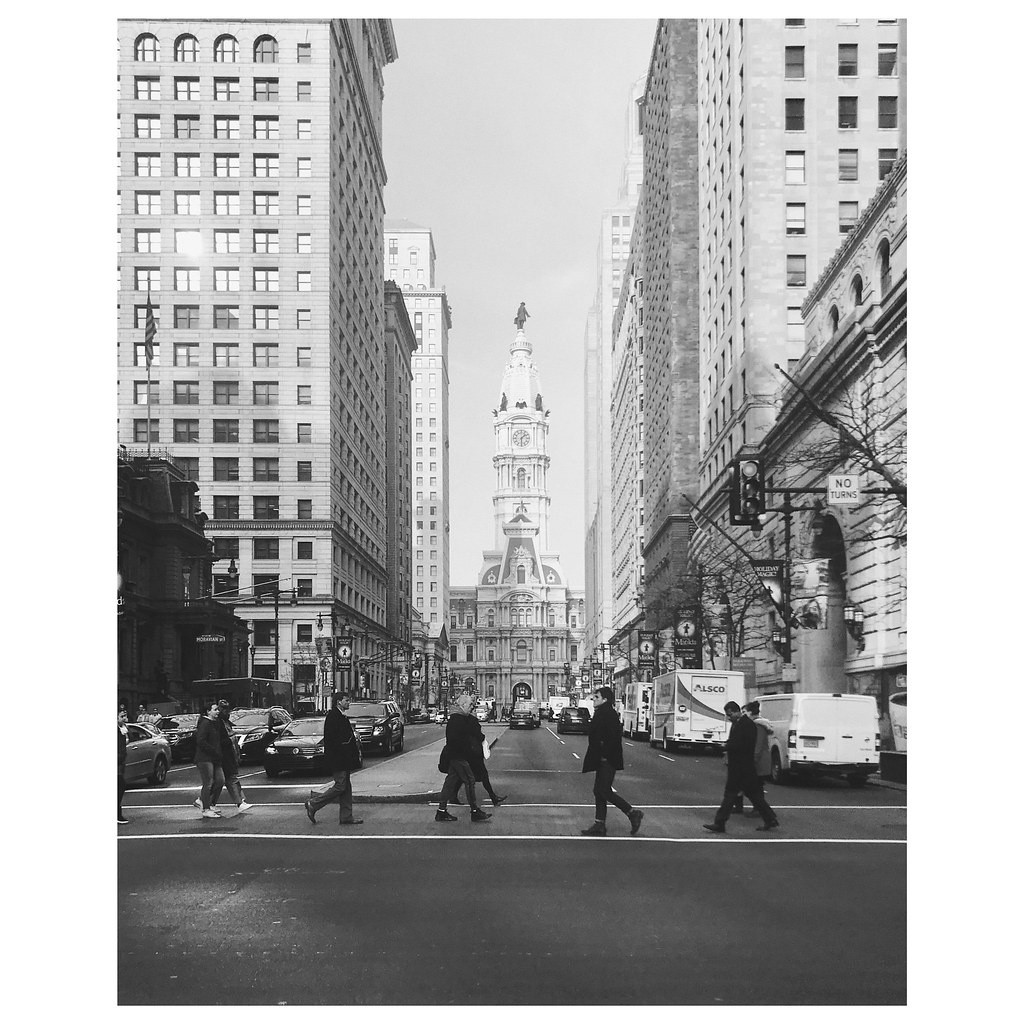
482 739 491 759
437 744 447 773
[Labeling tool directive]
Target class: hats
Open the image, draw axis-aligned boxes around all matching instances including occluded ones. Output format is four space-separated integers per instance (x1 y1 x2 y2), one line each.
218 699 231 707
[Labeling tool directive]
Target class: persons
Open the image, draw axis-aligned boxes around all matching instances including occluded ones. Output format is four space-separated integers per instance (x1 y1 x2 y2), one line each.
703 701 779 832
209 698 253 815
448 704 509 805
435 694 493 821
193 703 225 818
581 687 644 837
729 701 775 817
305 692 364 825
118 705 129 825
134 704 163 724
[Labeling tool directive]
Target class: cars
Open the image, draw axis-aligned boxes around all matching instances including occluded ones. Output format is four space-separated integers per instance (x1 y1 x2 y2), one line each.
263 719 364 777
408 707 453 723
122 722 173 785
152 714 203 761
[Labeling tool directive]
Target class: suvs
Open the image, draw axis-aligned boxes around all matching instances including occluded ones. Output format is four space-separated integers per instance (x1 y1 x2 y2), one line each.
343 699 407 756
557 706 593 735
226 706 294 763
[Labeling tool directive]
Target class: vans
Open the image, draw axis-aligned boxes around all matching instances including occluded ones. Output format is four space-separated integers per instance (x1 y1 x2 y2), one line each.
474 698 498 721
509 700 543 729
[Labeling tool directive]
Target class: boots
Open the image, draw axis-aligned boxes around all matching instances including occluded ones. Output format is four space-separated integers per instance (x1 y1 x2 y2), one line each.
746 806 760 817
730 796 744 814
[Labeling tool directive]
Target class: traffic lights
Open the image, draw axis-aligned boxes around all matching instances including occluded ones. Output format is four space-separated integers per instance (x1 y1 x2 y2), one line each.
738 458 766 514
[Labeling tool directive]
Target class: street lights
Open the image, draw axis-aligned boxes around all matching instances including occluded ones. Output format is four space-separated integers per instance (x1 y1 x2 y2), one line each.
579 627 645 697
433 661 475 708
400 644 421 710
317 608 351 708
249 644 257 677
272 587 298 681
639 573 744 677
749 492 827 695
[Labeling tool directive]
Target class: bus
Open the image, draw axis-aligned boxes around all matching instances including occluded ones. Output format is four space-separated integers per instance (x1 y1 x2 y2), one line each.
192 677 294 717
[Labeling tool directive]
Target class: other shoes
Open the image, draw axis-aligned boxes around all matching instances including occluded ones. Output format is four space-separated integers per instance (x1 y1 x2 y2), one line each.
449 801 464 805
756 818 778 830
492 795 507 806
203 810 221 818
703 823 725 832
236 799 253 813
210 805 221 813
628 810 644 835
117 816 129 823
305 800 316 823
581 820 606 836
340 818 363 824
435 808 457 821
470 808 492 821
193 797 201 808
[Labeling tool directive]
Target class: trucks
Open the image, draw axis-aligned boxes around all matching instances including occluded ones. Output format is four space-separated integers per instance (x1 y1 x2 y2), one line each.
547 696 571 720
751 693 882 784
611 683 654 740
650 668 746 755
